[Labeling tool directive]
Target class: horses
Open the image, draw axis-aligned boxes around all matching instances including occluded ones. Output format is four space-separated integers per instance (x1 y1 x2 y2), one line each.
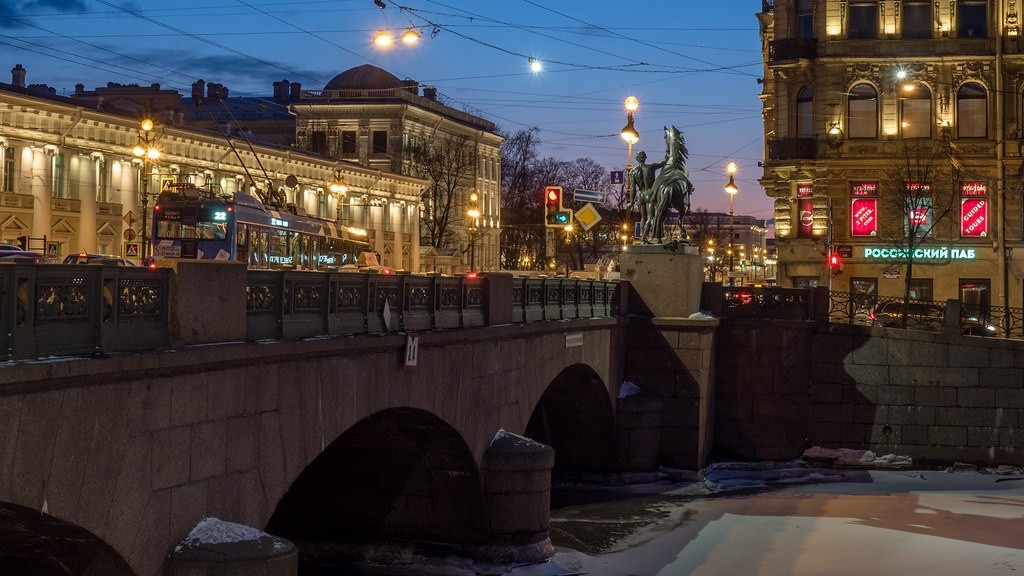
650 124 695 244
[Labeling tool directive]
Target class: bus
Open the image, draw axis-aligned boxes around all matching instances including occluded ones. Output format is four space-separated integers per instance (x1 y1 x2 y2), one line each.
151 183 380 271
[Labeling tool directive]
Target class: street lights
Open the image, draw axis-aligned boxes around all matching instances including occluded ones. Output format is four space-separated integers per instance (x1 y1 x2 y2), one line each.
134 119 159 265
725 163 739 286
564 226 573 278
330 163 383 224
621 96 639 245
468 193 478 272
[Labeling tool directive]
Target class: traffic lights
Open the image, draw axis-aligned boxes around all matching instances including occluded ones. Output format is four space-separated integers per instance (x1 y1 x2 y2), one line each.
741 264 745 267
545 186 573 227
831 256 838 265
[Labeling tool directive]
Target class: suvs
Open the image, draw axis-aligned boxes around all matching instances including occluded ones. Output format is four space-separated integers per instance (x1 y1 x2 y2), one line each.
62 247 135 267
874 300 997 336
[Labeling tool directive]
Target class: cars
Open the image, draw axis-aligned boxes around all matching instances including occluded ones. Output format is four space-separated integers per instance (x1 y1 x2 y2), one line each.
0 243 42 265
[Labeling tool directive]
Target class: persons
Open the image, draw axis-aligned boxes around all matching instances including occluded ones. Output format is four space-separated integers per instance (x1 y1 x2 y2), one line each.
626 151 666 244
252 230 268 268
